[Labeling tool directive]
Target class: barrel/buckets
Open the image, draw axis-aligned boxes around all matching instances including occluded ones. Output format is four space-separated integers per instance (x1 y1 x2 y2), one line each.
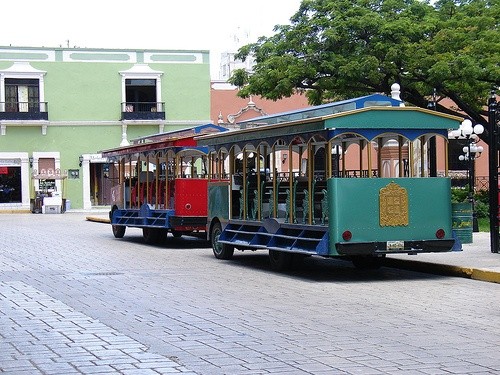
452 203 473 244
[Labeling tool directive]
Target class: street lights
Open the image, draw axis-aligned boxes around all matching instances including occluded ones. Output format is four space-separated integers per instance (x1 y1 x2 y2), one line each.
458 142 484 199
452 119 484 232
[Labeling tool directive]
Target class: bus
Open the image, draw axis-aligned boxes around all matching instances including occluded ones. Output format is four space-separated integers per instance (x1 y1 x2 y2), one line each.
97 123 231 241
193 93 467 272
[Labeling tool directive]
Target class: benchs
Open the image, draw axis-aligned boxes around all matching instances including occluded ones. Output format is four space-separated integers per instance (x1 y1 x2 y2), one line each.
240 169 379 225
132 171 229 210
